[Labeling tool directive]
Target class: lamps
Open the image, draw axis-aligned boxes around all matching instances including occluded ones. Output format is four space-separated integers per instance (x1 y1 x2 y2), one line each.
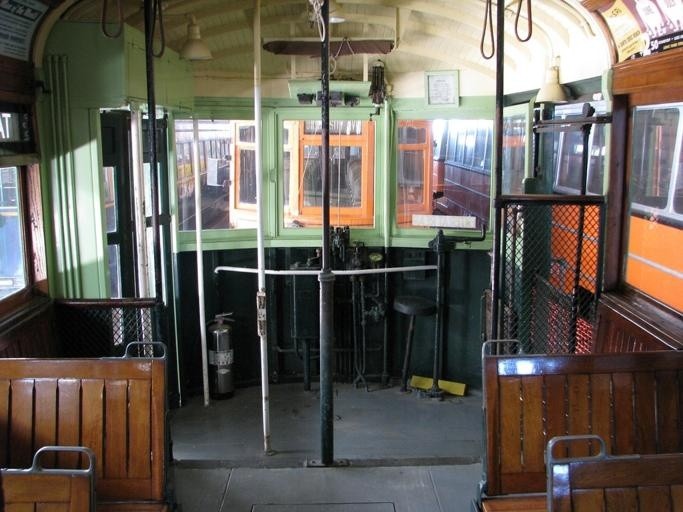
179 14 215 61
535 65 570 105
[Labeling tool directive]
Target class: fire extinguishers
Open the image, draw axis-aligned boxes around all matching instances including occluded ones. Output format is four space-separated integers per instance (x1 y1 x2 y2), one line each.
206 312 236 400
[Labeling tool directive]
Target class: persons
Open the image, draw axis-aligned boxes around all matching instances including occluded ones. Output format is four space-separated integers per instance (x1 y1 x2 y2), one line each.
303 145 345 189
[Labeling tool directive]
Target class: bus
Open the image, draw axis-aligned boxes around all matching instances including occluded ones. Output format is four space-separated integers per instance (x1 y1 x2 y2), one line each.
0 110 232 291
431 102 682 314
229 117 436 229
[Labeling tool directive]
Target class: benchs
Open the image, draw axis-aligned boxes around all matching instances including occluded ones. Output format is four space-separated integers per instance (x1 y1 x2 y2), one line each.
0 341 175 512
0 446 96 512
544 435 683 512
470 339 683 512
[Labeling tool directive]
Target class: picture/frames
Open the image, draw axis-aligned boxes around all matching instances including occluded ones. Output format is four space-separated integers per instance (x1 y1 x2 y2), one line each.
424 69 460 108
